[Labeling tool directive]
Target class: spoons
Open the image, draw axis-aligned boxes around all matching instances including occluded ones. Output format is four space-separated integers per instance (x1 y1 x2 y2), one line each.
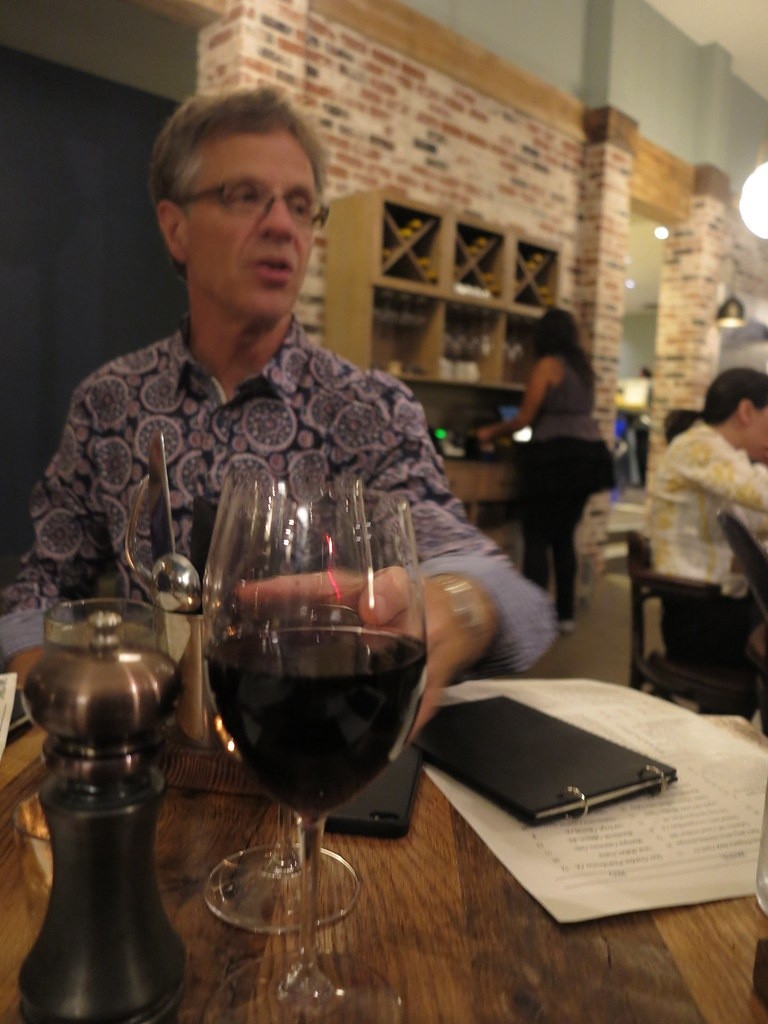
151 554 200 611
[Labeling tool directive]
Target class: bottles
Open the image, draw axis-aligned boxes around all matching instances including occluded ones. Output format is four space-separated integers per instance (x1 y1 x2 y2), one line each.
19 599 189 1023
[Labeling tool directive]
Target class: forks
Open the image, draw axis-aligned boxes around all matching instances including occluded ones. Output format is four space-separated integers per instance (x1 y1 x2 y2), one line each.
125 474 152 603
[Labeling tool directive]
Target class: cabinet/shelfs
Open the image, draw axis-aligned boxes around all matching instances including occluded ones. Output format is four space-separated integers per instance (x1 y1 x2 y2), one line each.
323 193 562 528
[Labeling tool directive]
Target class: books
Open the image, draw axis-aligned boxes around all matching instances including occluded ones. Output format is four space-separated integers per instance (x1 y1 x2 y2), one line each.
412 696 678 828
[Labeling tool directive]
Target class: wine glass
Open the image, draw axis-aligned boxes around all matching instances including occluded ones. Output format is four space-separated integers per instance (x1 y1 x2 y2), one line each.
203 474 428 1024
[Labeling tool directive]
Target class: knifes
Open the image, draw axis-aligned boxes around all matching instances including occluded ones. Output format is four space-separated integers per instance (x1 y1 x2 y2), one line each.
149 431 175 560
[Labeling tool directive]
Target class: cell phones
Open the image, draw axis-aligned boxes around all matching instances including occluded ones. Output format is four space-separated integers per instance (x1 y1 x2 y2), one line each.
325 743 420 839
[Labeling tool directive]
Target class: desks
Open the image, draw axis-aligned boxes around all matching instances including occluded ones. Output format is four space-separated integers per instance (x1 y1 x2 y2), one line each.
0 679 768 1024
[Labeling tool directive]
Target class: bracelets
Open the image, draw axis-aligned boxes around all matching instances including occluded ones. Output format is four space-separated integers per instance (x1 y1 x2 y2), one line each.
434 575 482 638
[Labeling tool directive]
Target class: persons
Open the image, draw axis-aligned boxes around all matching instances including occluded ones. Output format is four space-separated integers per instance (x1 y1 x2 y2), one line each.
649 368 768 672
479 310 602 623
0 86 558 740
633 368 651 483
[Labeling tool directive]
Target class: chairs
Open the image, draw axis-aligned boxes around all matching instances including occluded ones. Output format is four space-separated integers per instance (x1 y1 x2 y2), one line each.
626 530 749 716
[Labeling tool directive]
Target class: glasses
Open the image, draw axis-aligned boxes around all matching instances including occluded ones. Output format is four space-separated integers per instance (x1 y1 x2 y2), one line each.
174 176 329 227
